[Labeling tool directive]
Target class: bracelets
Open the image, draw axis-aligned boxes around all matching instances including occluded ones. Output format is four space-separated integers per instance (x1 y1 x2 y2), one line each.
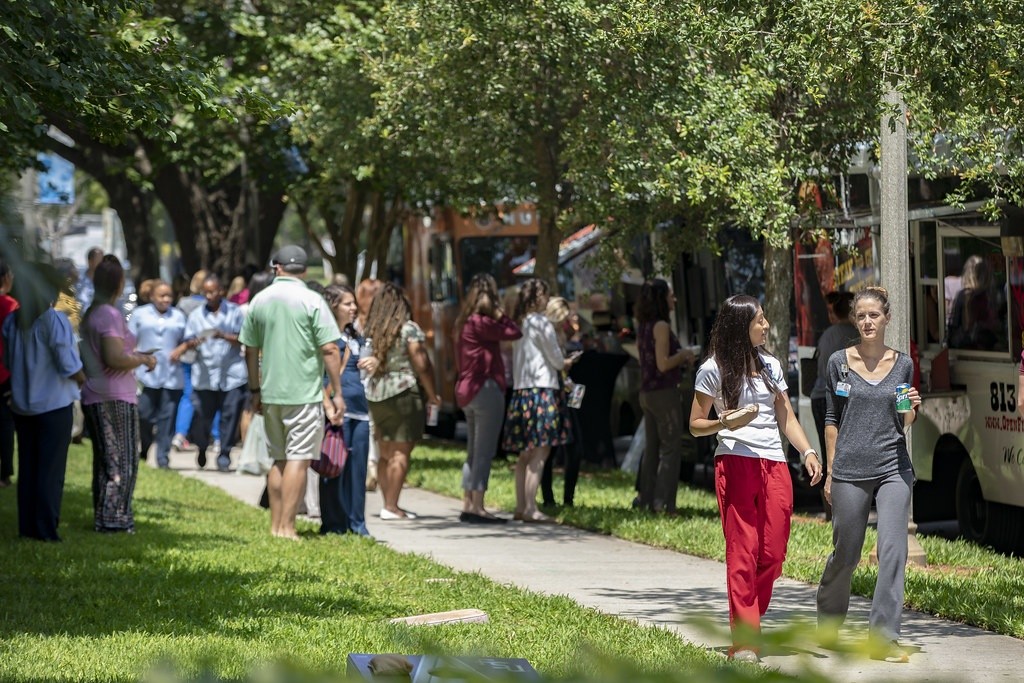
803 448 819 460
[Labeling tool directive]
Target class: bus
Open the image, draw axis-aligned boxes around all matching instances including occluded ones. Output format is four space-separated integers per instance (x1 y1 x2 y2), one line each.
651 166 1024 547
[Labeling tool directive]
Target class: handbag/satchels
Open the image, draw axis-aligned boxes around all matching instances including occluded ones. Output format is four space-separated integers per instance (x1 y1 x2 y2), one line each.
620 416 646 475
309 422 348 479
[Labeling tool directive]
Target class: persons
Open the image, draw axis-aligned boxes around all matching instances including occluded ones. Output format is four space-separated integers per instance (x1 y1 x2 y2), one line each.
688 293 824 668
939 248 1010 352
0 245 443 544
453 272 524 525
631 277 700 517
236 245 347 543
500 277 586 524
815 281 922 664
76 254 159 535
1017 349 1024 423
363 282 443 521
809 291 861 524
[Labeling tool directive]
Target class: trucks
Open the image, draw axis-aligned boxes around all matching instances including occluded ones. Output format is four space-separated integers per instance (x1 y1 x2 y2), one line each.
368 195 628 439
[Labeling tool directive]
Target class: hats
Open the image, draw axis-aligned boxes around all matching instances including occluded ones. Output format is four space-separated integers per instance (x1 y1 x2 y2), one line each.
272 245 308 265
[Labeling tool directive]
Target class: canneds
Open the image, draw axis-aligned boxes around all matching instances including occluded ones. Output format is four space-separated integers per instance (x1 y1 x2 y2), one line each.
426 403 438 426
896 383 911 412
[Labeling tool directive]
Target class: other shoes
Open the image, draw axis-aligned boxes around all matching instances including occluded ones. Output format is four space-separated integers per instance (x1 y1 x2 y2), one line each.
460 511 509 525
198 449 207 468
217 455 231 472
380 507 417 520
733 649 759 663
869 648 909 663
172 433 188 451
513 509 559 524
816 622 839 648
